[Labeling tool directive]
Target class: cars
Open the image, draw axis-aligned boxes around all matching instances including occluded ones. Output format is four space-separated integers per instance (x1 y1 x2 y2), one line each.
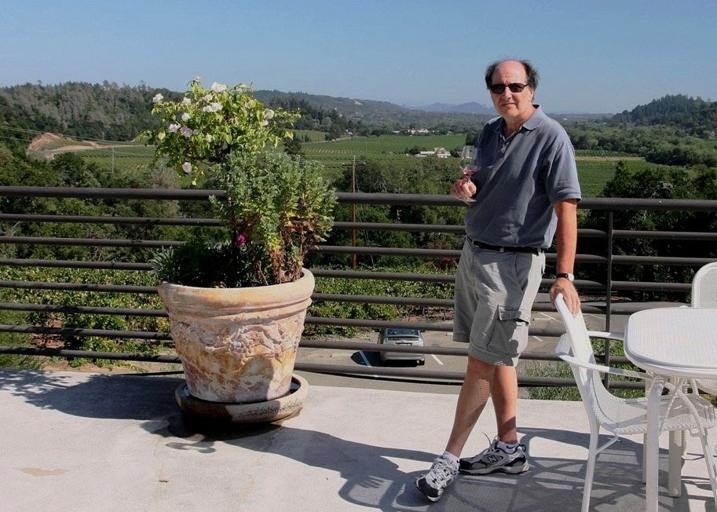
378 328 425 367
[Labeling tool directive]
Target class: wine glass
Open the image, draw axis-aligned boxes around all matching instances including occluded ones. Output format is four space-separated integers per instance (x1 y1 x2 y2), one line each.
460 145 483 204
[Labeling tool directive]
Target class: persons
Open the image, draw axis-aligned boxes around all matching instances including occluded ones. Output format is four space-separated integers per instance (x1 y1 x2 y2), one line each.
415 58 582 502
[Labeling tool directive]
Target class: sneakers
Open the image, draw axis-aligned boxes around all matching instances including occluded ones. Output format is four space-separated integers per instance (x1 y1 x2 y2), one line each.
460 438 530 475
415 453 461 503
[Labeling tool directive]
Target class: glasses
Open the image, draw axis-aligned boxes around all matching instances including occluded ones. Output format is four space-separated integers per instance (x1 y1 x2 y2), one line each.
488 83 530 94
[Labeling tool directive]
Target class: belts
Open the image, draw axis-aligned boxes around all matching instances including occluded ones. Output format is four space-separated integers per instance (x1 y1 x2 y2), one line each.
466 234 546 254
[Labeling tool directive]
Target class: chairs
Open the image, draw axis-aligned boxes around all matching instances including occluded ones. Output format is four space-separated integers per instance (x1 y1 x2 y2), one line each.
550 261 717 510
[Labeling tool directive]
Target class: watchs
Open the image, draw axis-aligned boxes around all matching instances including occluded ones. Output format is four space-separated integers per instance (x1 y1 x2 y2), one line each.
556 272 574 282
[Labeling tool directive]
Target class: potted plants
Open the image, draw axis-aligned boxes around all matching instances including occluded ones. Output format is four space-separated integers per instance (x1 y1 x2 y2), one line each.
142 74 338 425
